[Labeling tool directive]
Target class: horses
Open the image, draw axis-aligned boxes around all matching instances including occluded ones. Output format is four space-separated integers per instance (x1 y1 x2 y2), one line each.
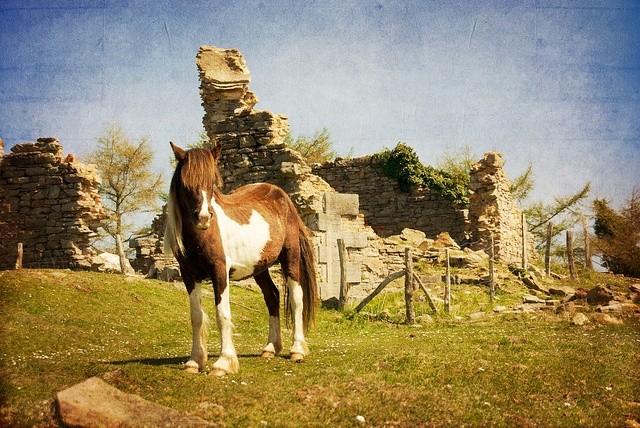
163 140 320 377
